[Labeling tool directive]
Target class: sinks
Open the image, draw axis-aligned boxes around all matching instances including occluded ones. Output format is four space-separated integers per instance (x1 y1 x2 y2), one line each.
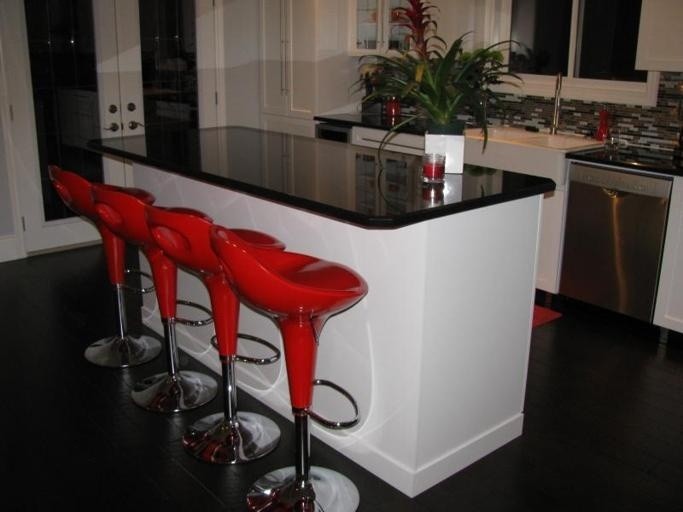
520 130 598 151
464 123 545 142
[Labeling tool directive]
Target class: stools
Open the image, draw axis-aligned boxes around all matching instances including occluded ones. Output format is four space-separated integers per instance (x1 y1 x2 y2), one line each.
49 165 164 367
89 181 217 416
144 205 286 474
207 224 369 511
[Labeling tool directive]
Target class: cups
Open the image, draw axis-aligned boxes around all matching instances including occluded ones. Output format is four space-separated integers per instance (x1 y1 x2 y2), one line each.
422 153 446 184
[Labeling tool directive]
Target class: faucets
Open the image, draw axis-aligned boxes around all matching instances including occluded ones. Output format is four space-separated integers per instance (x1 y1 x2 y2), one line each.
480 91 490 122
549 71 564 135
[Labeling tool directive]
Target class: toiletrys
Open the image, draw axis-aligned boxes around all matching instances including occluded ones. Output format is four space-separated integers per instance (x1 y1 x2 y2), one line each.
596 103 608 138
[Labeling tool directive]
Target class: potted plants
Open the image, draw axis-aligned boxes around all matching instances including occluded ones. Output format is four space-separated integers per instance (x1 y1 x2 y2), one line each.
352 0 529 174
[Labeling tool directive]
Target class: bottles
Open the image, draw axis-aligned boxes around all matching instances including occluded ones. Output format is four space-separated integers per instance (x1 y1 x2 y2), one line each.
597 108 608 141
387 96 401 116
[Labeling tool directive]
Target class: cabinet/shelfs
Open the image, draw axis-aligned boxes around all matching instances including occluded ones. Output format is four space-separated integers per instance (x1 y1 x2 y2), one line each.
348 0 414 58
257 0 317 121
